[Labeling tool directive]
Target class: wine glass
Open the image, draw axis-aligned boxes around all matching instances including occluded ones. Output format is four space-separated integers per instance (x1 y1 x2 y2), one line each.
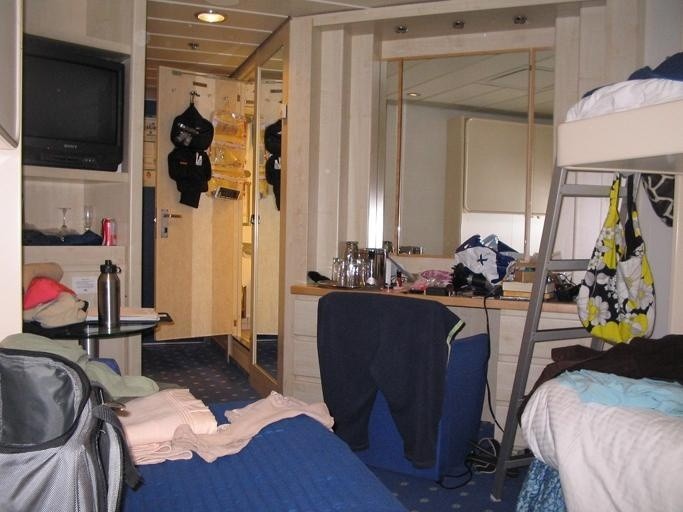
58 205 95 241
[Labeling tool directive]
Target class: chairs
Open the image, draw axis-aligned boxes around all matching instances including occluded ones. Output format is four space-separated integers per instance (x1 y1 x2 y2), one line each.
318 291 490 483
1 333 144 511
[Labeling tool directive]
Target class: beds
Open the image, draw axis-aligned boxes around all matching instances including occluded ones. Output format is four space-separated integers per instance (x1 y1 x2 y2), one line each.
516 102 683 512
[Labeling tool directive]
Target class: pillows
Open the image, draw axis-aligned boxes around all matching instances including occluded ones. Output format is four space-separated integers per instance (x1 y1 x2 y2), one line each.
563 76 683 122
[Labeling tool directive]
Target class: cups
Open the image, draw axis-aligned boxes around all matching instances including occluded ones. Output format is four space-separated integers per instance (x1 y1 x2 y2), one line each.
331 241 365 288
362 248 387 288
399 245 424 256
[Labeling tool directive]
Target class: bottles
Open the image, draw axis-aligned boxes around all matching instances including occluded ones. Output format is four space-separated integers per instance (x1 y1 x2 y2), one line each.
96 259 122 330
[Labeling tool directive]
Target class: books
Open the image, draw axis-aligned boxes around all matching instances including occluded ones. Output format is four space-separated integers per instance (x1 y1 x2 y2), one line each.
83 307 160 322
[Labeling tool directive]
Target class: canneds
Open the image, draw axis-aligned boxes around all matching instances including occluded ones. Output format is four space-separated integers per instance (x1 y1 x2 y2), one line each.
102 217 118 245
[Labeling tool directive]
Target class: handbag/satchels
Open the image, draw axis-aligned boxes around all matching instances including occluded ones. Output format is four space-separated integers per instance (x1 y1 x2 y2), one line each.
0 346 124 512
575 205 656 344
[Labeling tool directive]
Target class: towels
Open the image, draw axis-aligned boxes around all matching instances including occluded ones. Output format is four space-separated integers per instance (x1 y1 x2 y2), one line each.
558 369 683 416
171 392 335 464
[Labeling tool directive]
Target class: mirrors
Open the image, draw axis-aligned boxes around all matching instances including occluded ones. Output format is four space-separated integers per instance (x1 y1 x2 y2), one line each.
376 51 555 261
257 46 284 385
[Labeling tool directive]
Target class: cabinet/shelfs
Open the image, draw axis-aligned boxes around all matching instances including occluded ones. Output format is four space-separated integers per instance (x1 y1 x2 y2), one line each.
442 115 554 258
290 283 612 455
23 0 146 375
